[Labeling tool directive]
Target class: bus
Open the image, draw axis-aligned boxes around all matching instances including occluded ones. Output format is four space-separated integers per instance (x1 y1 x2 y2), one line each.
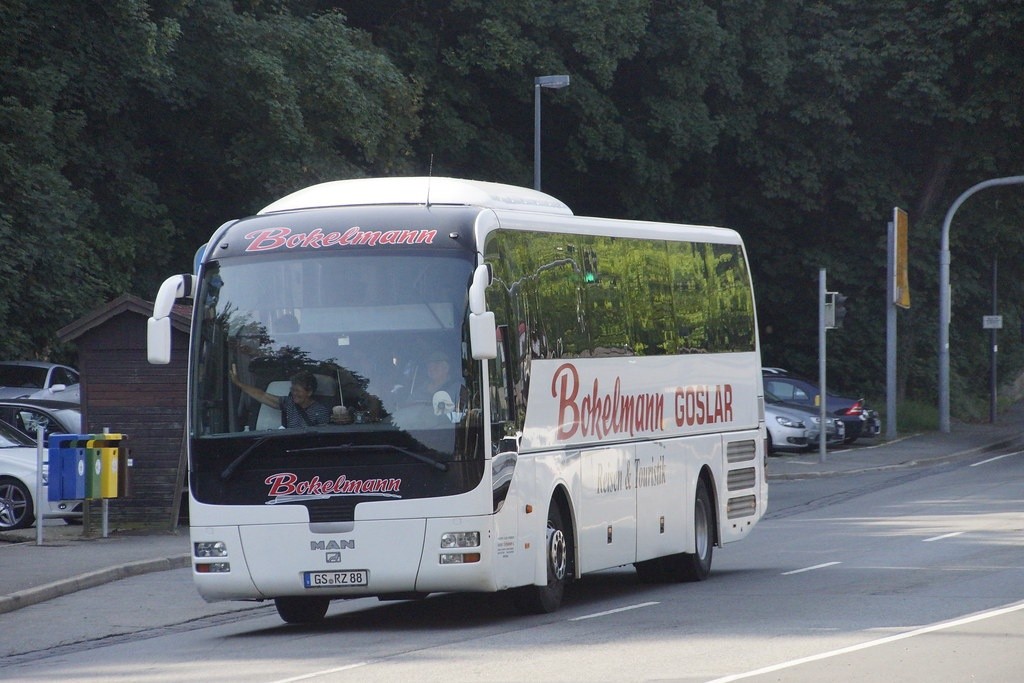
144 173 771 625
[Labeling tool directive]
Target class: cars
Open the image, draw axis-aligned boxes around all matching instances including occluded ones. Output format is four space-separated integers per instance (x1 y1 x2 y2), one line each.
0 419 86 530
764 389 846 454
0 357 82 444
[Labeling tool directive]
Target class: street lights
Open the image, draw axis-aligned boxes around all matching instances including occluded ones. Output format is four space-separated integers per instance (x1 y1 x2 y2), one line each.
532 74 573 193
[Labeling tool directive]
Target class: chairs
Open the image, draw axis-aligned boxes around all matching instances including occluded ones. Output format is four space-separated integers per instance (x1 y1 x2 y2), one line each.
256 374 338 431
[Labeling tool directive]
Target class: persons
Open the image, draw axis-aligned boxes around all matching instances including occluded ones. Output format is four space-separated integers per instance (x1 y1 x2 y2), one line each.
230 363 330 427
411 351 468 411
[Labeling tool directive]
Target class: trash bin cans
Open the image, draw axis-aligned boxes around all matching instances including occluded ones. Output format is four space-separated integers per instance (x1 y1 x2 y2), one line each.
46 431 130 502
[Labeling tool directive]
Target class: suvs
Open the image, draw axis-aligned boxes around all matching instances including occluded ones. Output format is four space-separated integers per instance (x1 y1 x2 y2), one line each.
761 367 882 444
